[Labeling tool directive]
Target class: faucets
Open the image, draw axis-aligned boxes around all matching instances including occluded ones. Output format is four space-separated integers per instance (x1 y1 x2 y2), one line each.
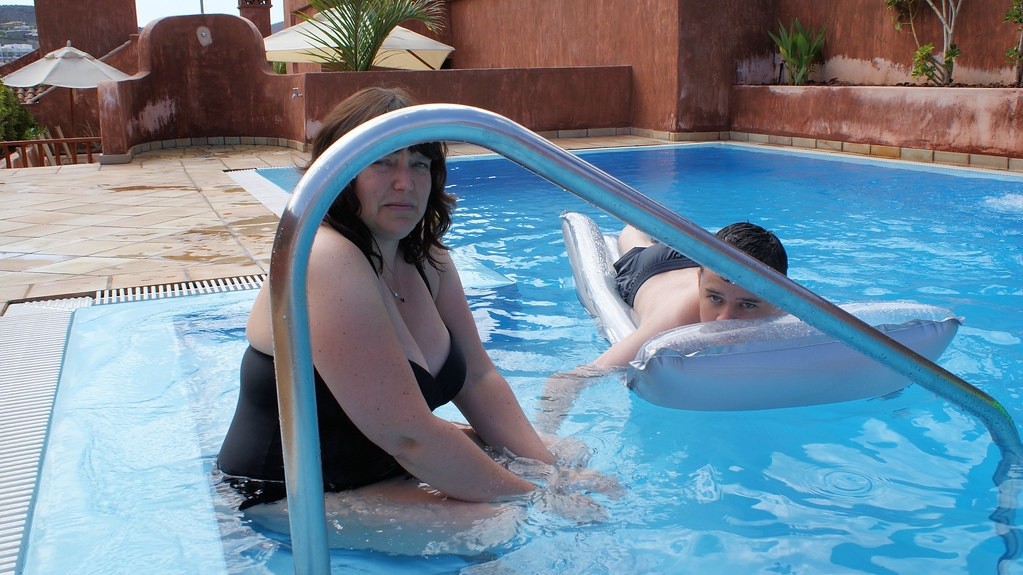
291 87 303 100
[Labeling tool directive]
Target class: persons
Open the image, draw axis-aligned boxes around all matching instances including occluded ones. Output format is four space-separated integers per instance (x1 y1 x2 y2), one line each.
217 85 555 500
590 221 790 369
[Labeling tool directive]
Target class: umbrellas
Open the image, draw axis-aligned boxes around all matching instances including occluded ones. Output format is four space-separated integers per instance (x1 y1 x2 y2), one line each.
263 0 455 70
0 38 135 123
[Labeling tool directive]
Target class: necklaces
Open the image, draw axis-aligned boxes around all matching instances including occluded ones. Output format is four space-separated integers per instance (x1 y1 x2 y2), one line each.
375 257 406 302
